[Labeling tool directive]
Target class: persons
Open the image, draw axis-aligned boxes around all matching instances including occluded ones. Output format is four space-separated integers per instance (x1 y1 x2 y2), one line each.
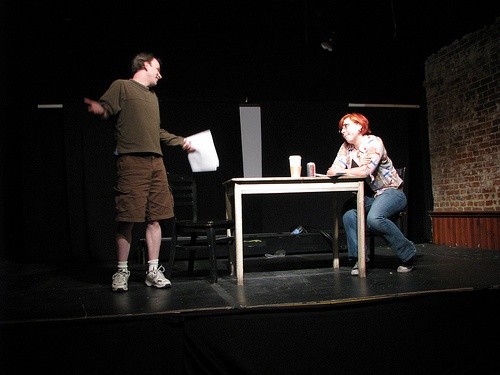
83 52 196 293
326 113 418 276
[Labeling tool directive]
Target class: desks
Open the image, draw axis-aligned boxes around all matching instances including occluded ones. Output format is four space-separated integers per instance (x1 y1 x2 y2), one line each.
222 175 367 284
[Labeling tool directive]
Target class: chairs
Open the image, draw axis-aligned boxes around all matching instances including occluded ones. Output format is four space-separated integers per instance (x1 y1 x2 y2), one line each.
369 166 408 264
168 173 235 284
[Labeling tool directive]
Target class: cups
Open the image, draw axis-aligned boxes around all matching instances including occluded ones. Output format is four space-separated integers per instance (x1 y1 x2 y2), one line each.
289 155 302 179
227 229 231 237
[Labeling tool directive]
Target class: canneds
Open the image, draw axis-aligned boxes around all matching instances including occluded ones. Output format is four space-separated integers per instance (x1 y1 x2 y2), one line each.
307 162 316 178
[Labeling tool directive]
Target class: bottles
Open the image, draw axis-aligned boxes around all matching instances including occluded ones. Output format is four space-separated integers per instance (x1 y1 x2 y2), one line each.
289 225 303 234
229 262 234 275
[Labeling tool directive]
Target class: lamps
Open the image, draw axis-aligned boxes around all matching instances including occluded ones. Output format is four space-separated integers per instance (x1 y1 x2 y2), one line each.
319 20 338 52
